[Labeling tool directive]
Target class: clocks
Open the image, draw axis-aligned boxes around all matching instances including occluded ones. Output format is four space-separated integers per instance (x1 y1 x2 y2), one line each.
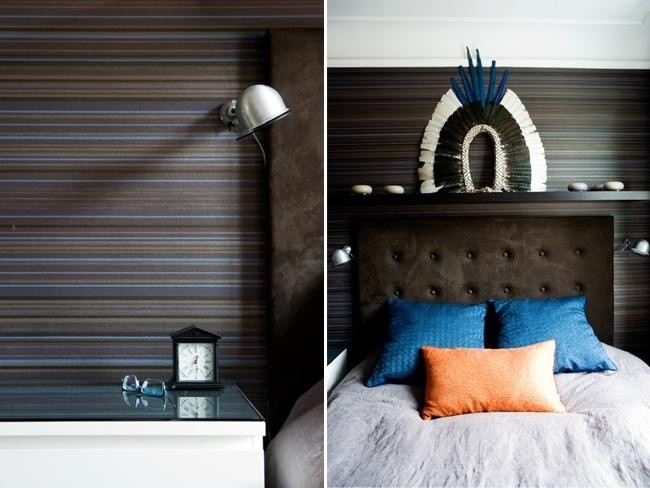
165 323 225 391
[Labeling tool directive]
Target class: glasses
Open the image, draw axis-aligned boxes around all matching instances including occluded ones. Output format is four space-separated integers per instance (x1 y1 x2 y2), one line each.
122 374 176 397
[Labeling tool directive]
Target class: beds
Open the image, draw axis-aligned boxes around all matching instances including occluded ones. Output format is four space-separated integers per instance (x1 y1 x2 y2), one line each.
264 214 649 487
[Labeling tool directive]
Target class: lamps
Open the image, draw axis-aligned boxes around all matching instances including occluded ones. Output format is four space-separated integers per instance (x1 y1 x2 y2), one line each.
621 237 650 256
221 85 292 141
331 245 354 267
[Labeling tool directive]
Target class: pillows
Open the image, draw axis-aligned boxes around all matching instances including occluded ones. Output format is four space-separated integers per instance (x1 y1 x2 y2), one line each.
418 340 566 421
488 295 618 374
366 294 488 388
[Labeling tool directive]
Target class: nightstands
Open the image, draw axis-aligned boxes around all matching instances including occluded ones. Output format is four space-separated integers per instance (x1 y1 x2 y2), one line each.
0 378 267 488
